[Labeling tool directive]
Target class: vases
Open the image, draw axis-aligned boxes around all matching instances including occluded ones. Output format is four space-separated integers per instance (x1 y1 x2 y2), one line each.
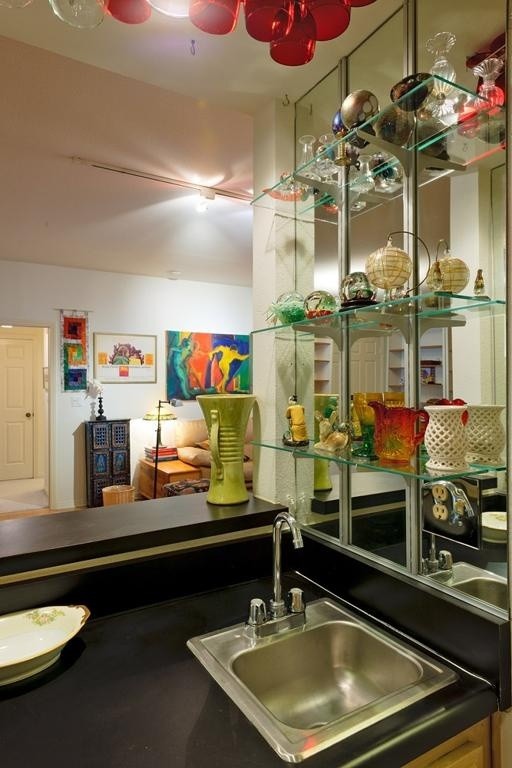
310 391 340 494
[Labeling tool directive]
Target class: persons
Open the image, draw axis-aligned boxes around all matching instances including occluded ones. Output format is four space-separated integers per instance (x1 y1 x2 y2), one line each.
284 394 309 441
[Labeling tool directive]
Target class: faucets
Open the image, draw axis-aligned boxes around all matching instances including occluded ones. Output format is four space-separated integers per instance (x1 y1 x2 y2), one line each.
425 481 477 526
268 511 304 618
427 530 439 573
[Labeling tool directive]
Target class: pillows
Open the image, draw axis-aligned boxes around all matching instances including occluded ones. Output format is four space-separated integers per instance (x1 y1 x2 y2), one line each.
176 447 211 467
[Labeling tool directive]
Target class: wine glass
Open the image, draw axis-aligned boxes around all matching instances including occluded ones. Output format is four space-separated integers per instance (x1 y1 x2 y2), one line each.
353 392 381 459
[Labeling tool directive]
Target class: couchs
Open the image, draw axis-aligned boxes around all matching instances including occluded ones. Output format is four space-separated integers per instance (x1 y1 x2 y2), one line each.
178 419 254 484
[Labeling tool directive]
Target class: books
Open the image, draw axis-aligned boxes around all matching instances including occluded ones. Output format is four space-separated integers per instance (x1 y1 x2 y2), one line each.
145 446 178 463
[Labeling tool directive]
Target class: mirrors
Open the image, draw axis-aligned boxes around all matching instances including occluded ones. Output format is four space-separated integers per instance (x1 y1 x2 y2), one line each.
295 0 507 627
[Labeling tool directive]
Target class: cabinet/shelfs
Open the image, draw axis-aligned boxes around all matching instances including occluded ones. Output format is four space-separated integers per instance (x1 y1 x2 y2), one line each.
313 325 449 403
84 418 134 507
360 696 511 768
139 459 203 500
295 100 506 488
250 76 489 489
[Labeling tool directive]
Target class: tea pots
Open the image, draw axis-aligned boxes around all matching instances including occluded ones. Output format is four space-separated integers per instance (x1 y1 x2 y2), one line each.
368 400 428 463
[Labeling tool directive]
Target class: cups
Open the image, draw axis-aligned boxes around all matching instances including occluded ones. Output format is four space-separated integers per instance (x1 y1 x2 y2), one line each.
0 0 376 68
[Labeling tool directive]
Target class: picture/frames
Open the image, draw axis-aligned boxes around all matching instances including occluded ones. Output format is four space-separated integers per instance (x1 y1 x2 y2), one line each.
163 329 253 405
91 331 158 384
57 306 94 394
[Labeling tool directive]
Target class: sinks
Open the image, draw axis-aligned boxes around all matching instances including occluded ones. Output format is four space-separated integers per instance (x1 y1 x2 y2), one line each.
435 561 508 614
185 596 461 765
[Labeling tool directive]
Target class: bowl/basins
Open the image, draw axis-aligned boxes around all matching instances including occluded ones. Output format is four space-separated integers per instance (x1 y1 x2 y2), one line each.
481 511 509 543
0 603 89 687
270 302 305 324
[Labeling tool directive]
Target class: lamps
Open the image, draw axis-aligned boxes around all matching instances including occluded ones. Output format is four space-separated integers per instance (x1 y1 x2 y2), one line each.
193 187 216 215
5 0 380 67
142 401 177 449
88 377 106 420
152 398 183 499
